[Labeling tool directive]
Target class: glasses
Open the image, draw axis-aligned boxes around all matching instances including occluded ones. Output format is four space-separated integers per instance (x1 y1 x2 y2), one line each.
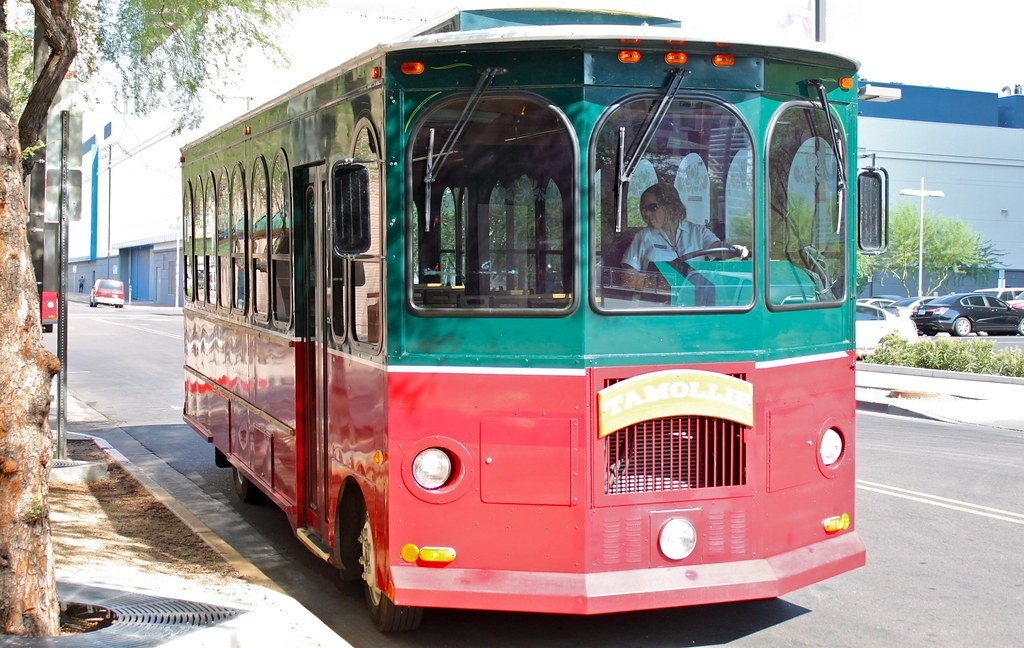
642 202 663 212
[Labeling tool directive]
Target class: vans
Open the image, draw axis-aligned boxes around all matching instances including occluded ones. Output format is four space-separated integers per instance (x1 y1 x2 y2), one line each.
973 287 1024 337
89 278 126 309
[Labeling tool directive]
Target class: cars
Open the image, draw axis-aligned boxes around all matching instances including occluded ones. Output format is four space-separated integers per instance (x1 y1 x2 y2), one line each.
855 301 918 355
909 292 1024 338
886 296 936 320
780 294 818 304
872 295 904 301
856 298 896 309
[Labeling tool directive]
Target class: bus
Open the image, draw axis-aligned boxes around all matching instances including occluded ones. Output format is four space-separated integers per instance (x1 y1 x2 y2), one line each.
42 222 58 332
177 9 871 639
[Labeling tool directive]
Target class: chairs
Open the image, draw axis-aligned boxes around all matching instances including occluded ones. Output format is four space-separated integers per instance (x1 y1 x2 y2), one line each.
606 229 639 286
412 283 571 309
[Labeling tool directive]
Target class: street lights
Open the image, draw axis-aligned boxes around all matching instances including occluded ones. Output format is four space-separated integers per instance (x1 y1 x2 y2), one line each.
898 176 945 298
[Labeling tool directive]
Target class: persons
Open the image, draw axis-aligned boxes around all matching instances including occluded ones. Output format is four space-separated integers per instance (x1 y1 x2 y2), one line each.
621 182 748 291
78 275 85 293
